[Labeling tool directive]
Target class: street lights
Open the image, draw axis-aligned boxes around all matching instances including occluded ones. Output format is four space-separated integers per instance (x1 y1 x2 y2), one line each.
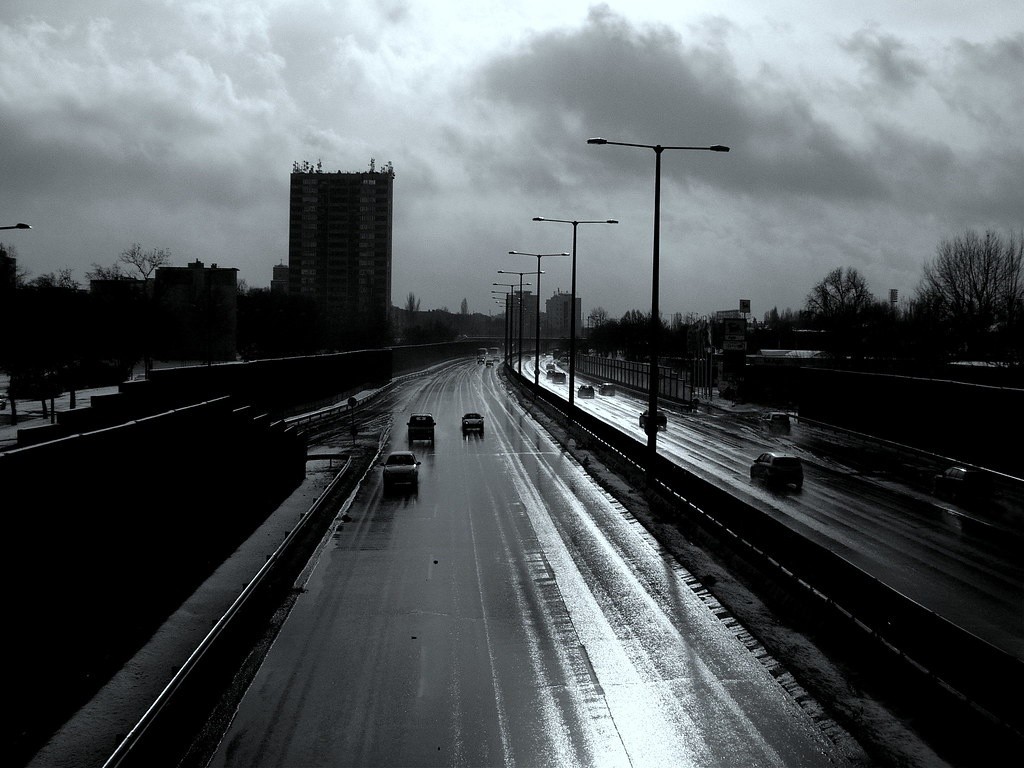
585 137 730 471
509 251 570 390
491 270 545 367
533 217 619 418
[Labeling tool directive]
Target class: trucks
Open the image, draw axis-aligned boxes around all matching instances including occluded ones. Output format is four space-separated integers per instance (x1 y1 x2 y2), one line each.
406 412 436 446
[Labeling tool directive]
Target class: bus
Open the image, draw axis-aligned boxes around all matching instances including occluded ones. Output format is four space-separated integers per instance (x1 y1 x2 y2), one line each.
477 348 488 357
489 347 499 355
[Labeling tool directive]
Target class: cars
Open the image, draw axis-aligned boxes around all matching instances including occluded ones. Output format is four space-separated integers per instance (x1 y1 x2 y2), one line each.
486 359 494 366
639 409 667 429
546 363 556 370
934 467 983 496
462 413 485 438
758 412 791 432
578 385 595 399
493 357 500 362
598 383 615 396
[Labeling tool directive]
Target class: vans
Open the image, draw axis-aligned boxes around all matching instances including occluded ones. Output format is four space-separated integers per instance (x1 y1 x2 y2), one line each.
381 451 421 492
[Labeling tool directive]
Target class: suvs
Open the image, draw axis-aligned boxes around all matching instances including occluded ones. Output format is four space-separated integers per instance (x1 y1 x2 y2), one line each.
750 451 803 490
477 355 485 364
547 370 566 384
